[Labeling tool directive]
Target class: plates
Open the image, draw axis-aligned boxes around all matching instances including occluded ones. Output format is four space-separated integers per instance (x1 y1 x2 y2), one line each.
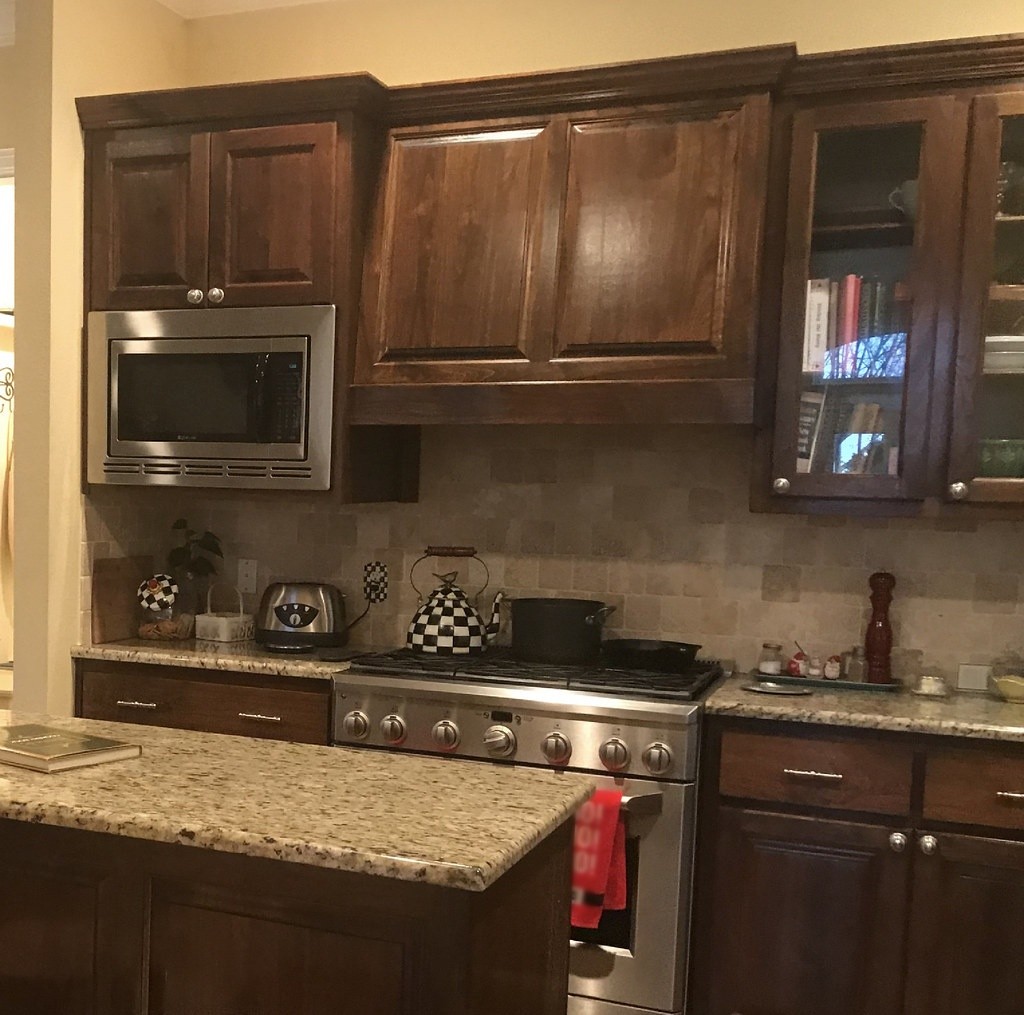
740 682 814 695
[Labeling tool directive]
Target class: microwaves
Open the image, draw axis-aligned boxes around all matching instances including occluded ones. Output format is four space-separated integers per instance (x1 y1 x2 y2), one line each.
86 304 337 491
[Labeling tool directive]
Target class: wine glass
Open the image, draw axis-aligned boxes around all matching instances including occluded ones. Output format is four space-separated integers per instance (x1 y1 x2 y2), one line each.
995 160 1018 217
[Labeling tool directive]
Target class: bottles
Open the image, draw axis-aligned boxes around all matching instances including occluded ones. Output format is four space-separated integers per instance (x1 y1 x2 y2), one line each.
758 642 783 675
847 647 869 683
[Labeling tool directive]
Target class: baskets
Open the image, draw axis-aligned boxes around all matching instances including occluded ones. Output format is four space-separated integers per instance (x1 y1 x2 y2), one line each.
195 583 254 642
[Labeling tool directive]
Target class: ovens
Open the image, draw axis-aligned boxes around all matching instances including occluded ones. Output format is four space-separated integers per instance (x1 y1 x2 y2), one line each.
525 766 695 1015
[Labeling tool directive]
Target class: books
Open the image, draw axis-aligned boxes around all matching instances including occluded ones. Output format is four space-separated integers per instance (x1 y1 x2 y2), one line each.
0 724 142 774
796 392 899 475
801 274 896 372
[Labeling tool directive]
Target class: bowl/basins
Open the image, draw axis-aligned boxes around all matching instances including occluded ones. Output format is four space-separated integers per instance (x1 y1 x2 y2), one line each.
992 674 1024 704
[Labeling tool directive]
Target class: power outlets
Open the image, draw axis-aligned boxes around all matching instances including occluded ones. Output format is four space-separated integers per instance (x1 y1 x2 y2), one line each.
363 561 387 604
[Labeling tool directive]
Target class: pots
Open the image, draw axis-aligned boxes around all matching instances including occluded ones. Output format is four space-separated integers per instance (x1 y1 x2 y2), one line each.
511 597 617 667
601 639 703 676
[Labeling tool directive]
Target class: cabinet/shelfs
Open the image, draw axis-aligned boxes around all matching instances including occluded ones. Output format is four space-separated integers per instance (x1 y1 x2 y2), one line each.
745 31 1024 523
76 71 421 505
349 41 797 428
688 715 1023 1015
74 656 333 746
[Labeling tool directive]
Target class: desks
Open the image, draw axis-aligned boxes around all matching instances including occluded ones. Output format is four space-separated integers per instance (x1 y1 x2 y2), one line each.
0 707 592 1015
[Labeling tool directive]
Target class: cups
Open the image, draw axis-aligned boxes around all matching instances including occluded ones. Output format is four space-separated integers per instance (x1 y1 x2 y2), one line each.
919 676 947 696
888 180 918 222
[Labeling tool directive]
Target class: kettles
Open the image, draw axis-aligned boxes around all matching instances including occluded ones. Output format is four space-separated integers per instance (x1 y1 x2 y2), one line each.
405 545 505 659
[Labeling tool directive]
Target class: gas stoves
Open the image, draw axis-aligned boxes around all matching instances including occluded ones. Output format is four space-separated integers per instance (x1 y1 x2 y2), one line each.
328 647 724 780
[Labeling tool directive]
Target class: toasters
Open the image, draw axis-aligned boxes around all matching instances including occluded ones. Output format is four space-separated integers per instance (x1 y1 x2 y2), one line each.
254 582 350 648
